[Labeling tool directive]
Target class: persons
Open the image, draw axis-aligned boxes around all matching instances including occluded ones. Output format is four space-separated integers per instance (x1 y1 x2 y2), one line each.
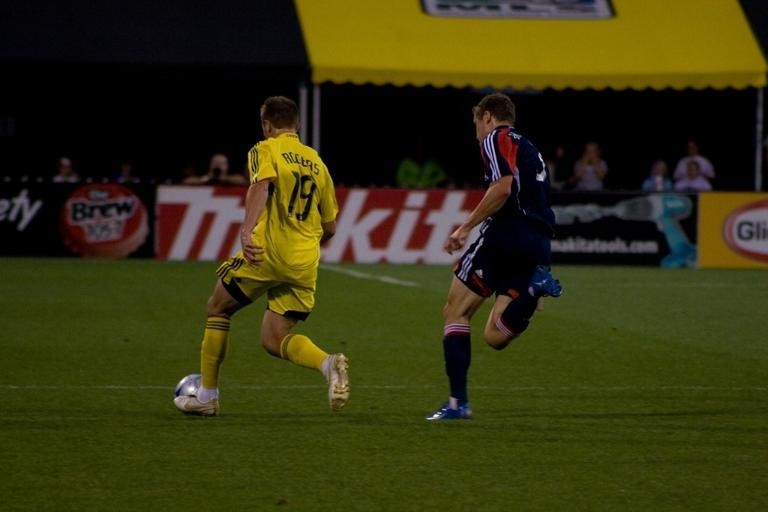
642 160 673 192
184 154 247 184
543 142 569 195
425 91 562 420
112 163 140 182
51 157 80 182
671 162 713 192
174 93 353 415
572 143 608 194
673 141 717 178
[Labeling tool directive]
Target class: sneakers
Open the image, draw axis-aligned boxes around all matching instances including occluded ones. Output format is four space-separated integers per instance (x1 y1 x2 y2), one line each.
529 263 562 299
170 394 221 418
324 351 352 413
423 399 472 423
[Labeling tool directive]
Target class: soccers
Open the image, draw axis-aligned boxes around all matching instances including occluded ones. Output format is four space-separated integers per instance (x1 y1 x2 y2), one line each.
175 374 201 397
